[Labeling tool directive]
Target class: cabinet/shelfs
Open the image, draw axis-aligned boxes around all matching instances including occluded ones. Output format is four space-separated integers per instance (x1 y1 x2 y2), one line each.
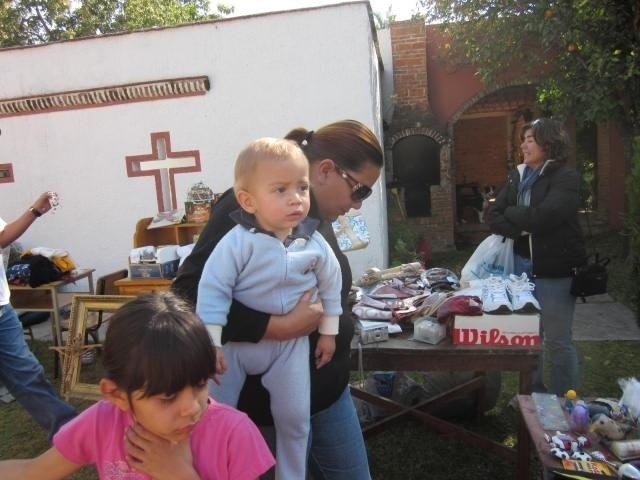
133 216 207 250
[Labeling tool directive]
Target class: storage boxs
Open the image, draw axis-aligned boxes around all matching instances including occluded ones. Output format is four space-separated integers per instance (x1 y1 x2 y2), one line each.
453 315 541 347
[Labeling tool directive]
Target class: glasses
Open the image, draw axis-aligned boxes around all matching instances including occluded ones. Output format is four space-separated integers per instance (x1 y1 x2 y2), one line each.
331 160 374 204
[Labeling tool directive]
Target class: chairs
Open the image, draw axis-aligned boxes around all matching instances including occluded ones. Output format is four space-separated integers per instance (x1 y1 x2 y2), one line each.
53 268 128 377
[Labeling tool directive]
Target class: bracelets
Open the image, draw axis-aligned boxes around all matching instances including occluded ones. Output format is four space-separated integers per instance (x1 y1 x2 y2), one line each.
27 207 42 217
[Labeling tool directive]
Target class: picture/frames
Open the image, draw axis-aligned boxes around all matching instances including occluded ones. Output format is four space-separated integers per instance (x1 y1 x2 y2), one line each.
60 294 133 400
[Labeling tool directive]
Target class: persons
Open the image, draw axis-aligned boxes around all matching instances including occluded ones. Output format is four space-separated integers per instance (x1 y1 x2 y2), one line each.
194 138 343 479
0 291 279 479
169 119 386 480
482 116 586 397
0 190 77 445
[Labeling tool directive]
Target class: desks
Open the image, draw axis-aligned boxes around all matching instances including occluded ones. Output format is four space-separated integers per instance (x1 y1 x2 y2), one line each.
114 275 175 301
9 266 103 379
457 182 482 210
514 390 639 480
349 275 537 480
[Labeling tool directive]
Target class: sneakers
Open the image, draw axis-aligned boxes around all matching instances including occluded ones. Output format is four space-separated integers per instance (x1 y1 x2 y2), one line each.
507 391 522 412
481 270 543 317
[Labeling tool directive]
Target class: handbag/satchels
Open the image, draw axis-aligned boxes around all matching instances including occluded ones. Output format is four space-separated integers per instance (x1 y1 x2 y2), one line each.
568 248 612 304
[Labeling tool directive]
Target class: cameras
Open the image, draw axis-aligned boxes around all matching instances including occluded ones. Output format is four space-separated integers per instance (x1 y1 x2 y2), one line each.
360 323 388 345
